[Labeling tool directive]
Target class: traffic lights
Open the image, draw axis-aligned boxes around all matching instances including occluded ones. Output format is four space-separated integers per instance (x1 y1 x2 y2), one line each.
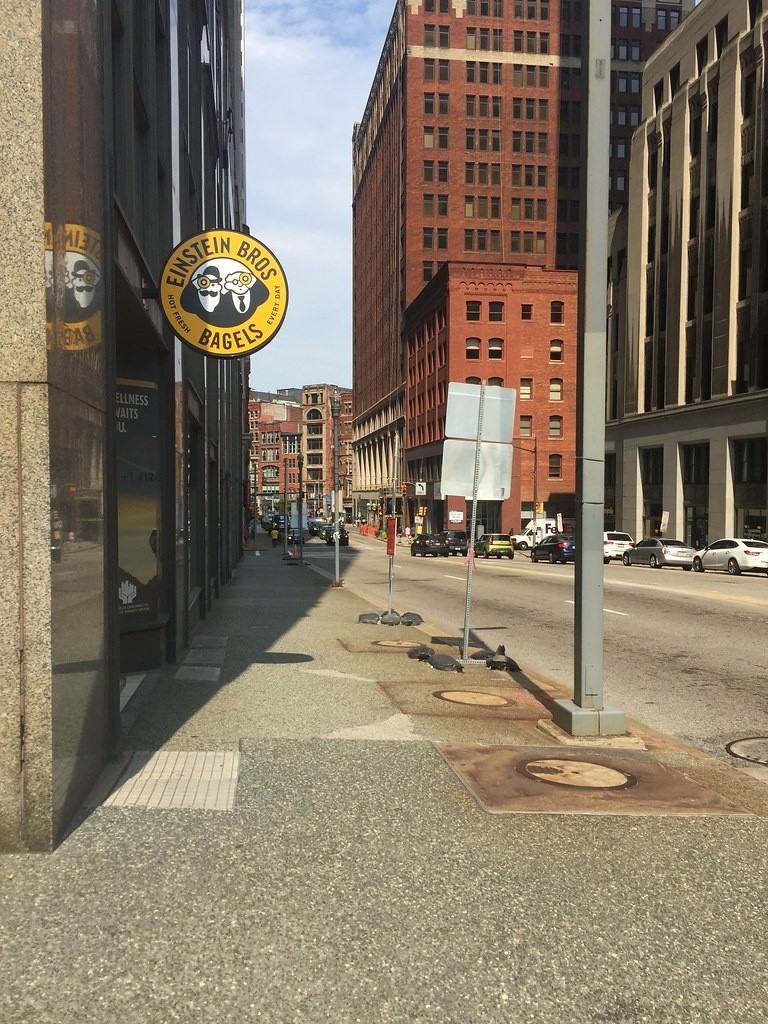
402 485 408 498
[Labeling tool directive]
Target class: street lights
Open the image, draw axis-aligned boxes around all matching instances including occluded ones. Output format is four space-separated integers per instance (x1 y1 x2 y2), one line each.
329 385 345 588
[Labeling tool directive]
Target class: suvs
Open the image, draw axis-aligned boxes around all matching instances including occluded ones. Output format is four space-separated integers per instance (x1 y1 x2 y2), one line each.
437 530 467 556
603 530 637 565
474 533 514 560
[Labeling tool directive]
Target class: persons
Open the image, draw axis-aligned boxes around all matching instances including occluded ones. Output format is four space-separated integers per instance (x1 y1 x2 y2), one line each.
271 528 278 548
244 524 254 539
405 526 416 540
355 518 366 526
509 528 513 536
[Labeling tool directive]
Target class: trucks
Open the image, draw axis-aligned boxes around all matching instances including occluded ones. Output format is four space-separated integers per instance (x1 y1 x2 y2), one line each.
509 518 576 550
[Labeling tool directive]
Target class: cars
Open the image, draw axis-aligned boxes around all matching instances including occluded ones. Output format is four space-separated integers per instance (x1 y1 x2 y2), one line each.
410 534 449 557
327 527 349 546
530 531 577 565
261 515 344 540
623 539 697 572
288 528 305 545
692 538 768 576
